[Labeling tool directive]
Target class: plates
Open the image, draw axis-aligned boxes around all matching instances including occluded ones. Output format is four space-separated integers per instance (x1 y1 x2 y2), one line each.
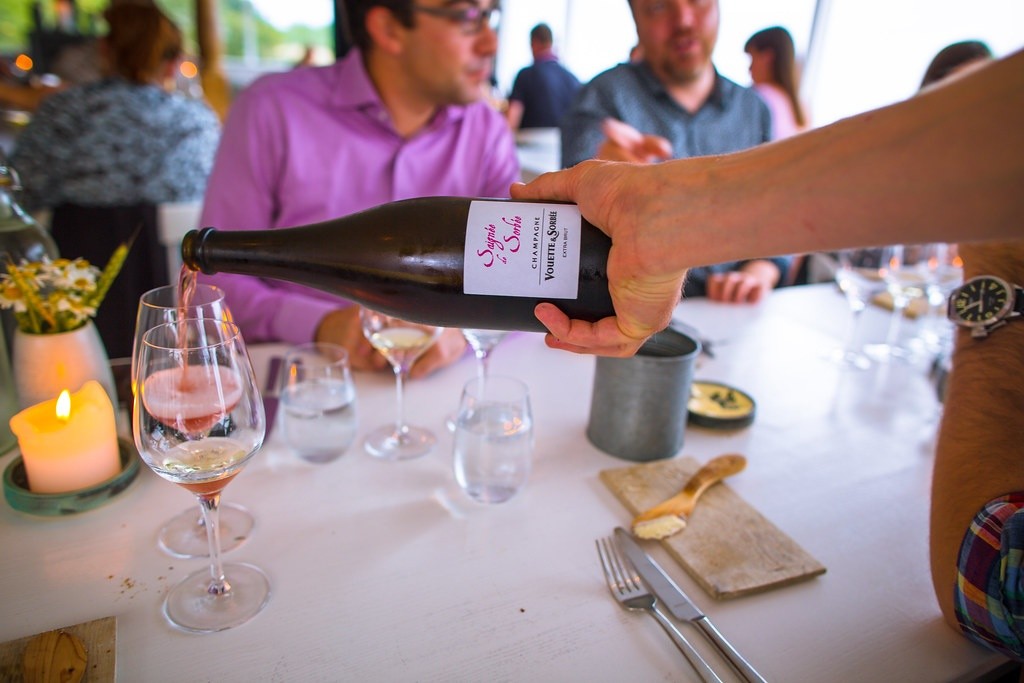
686 379 757 427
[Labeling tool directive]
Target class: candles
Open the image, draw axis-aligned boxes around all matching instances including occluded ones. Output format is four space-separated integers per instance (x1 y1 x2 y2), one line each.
6 380 122 495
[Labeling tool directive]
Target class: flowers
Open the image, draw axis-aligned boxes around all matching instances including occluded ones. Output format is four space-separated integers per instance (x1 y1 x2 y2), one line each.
0 245 129 335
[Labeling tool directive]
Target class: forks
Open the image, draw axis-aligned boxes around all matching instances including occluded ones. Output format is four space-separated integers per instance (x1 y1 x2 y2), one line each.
595 536 723 683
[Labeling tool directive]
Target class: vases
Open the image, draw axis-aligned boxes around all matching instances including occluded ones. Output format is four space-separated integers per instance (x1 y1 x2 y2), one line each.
13 318 121 431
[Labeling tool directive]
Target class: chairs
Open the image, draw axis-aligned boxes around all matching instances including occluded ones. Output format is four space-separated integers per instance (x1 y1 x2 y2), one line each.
46 199 175 359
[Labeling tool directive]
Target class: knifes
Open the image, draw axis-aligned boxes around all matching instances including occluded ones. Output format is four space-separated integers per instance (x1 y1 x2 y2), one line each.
614 526 769 683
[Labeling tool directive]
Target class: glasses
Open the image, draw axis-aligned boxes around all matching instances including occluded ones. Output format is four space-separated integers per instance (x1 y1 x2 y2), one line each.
393 3 502 35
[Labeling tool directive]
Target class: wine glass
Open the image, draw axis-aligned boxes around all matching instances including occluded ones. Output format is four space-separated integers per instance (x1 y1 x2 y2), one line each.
129 284 274 637
358 304 445 462
445 328 509 434
827 239 962 371
272 344 358 506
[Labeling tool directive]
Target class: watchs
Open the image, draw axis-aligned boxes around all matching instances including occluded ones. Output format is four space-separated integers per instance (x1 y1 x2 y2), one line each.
946 274 1023 339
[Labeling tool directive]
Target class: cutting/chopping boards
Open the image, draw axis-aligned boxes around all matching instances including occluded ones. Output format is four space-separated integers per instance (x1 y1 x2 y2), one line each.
600 455 827 602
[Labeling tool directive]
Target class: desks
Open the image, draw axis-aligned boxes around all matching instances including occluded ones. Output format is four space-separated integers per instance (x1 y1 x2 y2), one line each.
0 258 1014 682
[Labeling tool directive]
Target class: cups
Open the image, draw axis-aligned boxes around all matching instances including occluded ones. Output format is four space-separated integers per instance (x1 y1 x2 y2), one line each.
586 326 704 463
453 376 539 506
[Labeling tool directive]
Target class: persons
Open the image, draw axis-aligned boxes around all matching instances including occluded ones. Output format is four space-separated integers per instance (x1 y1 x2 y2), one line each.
805 40 991 284
928 236 1024 663
506 22 585 135
560 0 793 306
179 0 522 375
743 27 809 141
6 0 225 210
511 47 1024 359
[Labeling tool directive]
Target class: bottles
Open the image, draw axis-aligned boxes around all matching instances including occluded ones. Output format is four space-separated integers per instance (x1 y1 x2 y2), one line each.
0 166 62 280
181 195 616 333
